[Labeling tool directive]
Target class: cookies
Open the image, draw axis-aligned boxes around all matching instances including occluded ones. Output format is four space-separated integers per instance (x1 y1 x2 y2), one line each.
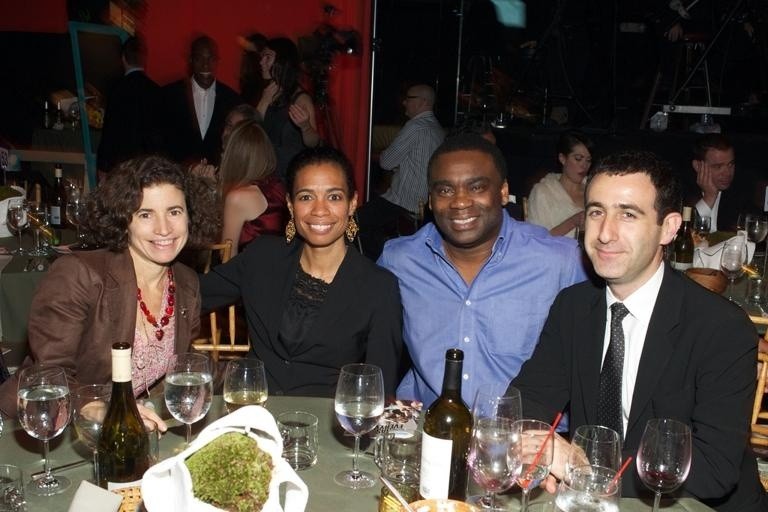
110 487 142 512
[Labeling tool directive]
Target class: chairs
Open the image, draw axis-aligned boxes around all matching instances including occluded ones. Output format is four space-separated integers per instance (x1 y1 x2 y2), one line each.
747 316 768 447
192 239 251 361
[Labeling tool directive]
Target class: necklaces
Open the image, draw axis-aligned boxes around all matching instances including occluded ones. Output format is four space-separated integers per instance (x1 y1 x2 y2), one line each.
135 262 177 341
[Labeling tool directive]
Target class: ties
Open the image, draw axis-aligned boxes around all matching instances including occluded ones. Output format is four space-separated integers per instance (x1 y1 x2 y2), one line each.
597 301 631 447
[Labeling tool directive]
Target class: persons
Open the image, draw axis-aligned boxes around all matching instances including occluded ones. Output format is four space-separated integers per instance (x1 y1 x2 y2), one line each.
197 137 402 408
495 144 768 512
162 37 240 165
345 84 446 258
373 131 596 439
683 137 755 234
527 130 595 238
255 37 317 170
190 105 290 261
96 36 162 169
0 155 202 441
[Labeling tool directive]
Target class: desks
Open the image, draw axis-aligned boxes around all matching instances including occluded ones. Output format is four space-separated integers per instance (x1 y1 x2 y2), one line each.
664 247 768 333
0 218 80 345
0 120 96 194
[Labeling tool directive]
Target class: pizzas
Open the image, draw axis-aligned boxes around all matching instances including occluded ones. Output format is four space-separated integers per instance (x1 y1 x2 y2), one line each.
383 400 424 425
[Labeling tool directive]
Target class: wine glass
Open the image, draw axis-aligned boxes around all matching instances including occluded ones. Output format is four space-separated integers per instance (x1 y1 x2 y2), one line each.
635 417 694 512
331 361 385 492
24 202 48 257
72 384 113 488
509 420 554 512
479 385 522 511
7 199 28 256
163 352 213 453
64 189 91 251
17 363 72 496
672 207 768 314
560 421 630 512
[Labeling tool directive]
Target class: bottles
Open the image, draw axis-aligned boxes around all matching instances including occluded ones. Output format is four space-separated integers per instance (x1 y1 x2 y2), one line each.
41 98 80 132
420 348 474 503
46 164 69 228
96 341 150 512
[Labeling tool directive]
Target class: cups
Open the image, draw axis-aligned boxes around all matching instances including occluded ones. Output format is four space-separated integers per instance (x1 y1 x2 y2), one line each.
276 411 319 477
140 418 160 468
223 358 270 415
0 464 26 512
372 423 419 493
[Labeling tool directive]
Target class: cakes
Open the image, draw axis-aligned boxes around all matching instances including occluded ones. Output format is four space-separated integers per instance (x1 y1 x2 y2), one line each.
185 431 272 512
